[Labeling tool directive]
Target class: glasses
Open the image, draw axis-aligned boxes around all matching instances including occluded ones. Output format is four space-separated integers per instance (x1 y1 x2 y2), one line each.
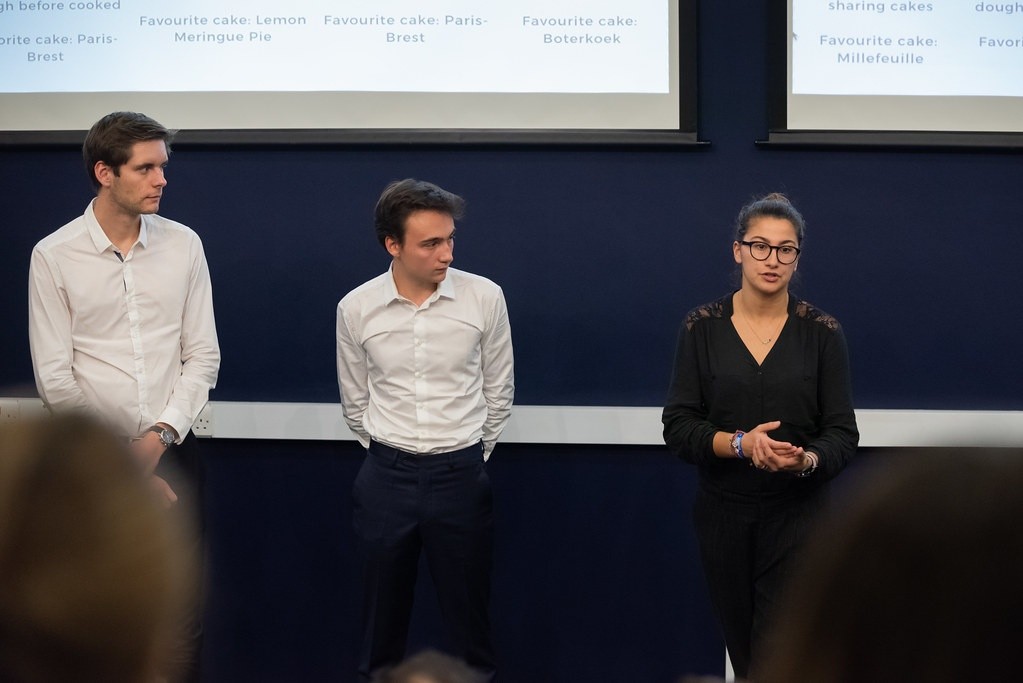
738 240 803 265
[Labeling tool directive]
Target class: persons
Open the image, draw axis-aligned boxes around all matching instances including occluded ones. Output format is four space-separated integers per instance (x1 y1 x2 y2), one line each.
333 180 516 683
26 112 222 682
659 191 861 682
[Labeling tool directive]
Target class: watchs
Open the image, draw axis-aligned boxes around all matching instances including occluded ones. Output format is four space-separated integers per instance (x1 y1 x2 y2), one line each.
791 453 818 478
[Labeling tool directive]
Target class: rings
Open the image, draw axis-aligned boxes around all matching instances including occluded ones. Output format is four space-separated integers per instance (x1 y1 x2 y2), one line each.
761 465 768 470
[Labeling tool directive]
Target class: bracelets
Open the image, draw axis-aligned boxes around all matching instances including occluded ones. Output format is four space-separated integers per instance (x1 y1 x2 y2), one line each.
729 429 747 460
